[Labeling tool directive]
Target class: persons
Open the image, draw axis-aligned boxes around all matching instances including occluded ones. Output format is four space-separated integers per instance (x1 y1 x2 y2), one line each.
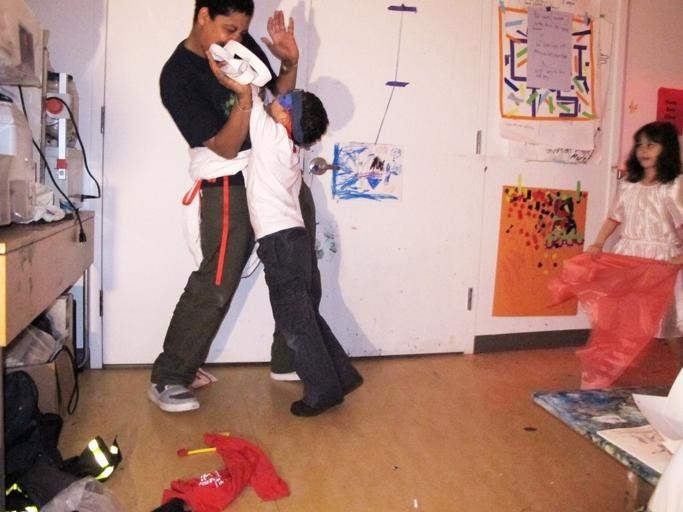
246 82 365 420
582 120 681 348
141 0 318 417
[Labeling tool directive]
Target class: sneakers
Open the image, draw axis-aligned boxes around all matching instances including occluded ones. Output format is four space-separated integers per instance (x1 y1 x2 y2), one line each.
270 367 363 416
146 380 199 413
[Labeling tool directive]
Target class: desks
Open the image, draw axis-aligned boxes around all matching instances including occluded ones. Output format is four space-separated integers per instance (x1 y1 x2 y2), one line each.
531 387 683 512
0 205 94 368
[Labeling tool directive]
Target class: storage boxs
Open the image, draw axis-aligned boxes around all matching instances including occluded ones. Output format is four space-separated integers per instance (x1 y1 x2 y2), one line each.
5 342 75 420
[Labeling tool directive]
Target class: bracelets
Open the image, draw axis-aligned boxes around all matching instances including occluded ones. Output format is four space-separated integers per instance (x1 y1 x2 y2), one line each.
279 62 298 73
234 96 251 114
